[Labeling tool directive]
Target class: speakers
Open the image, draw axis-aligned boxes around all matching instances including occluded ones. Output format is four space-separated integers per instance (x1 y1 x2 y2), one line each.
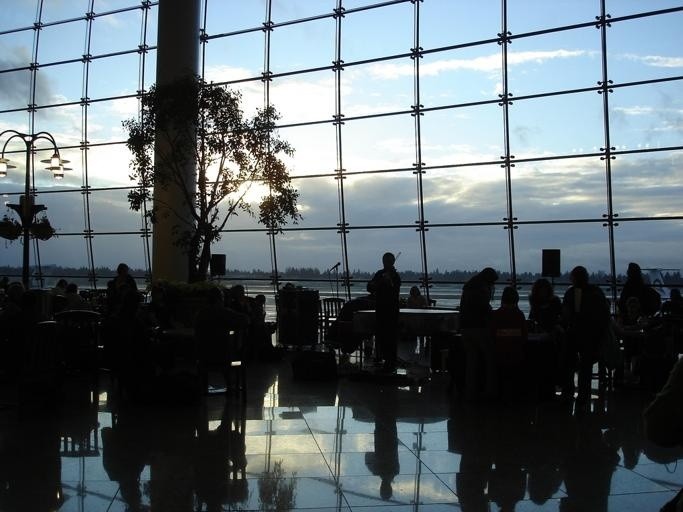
542 249 560 277
212 254 226 275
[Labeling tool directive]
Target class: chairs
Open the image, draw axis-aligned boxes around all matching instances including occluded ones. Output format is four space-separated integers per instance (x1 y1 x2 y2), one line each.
15 289 265 423
275 288 322 346
319 298 347 356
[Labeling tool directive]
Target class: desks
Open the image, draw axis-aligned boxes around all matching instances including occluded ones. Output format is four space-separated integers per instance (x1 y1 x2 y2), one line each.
353 309 624 418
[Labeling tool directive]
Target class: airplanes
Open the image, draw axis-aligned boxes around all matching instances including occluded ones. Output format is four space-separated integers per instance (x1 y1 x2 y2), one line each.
604 267 683 301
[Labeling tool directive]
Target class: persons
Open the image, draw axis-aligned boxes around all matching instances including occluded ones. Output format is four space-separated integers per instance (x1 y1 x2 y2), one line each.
407 285 427 309
0 262 276 377
447 261 682 399
372 390 400 501
366 249 401 369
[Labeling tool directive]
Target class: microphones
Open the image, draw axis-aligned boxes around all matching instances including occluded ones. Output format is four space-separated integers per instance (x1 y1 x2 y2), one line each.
330 262 340 271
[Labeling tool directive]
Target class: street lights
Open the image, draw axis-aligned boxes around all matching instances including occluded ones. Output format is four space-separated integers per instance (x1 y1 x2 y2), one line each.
0 130 73 290
